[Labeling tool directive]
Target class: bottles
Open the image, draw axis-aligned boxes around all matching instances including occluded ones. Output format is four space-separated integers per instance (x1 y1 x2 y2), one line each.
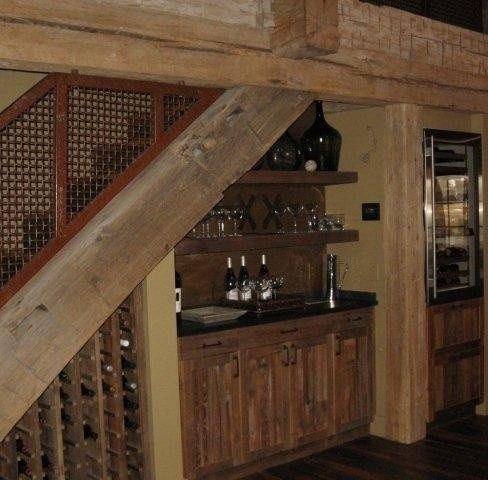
174 270 183 321
14 304 141 479
223 255 274 302
268 99 344 170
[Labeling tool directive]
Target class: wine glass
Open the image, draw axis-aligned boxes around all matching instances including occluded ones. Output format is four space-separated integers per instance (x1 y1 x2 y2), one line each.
235 271 285 313
185 204 244 240
271 199 320 235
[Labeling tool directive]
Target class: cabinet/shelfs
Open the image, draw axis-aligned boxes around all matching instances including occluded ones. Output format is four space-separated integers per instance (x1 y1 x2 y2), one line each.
428 299 484 419
337 305 376 431
173 167 361 253
240 313 336 465
179 330 241 479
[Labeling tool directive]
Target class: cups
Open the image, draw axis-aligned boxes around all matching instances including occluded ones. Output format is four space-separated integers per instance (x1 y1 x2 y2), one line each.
319 213 348 233
